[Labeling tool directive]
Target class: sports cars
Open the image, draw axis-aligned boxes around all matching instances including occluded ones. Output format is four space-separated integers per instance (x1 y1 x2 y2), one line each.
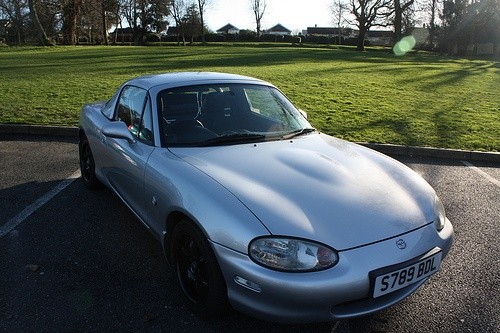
74 72 455 325
